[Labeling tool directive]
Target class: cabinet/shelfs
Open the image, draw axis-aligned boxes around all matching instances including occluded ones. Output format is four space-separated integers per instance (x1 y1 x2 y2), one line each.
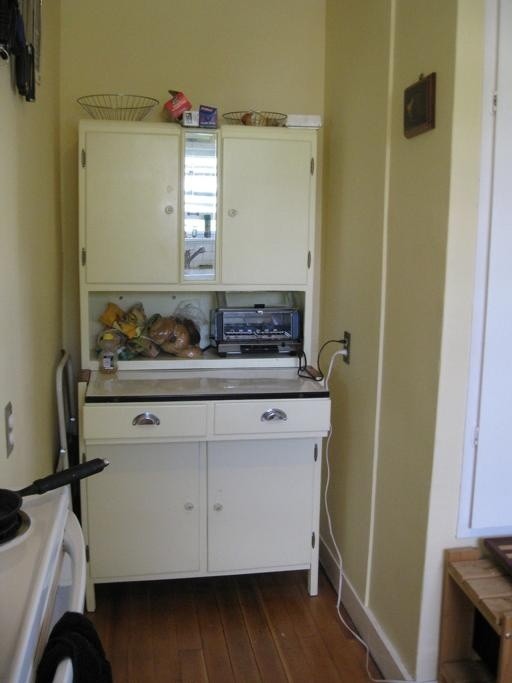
78 369 332 613
78 119 320 372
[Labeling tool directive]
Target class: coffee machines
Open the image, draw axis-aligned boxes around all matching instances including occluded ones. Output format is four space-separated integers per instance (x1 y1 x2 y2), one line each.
210 303 300 355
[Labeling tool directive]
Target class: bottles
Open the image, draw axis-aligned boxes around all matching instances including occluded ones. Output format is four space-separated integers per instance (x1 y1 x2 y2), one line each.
98 332 118 375
203 215 212 236
192 225 197 238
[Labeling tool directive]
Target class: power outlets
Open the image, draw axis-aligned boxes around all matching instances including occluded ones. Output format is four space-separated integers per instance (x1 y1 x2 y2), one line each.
343 331 351 364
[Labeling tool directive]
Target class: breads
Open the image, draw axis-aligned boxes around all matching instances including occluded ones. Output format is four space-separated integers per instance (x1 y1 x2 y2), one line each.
149 316 203 359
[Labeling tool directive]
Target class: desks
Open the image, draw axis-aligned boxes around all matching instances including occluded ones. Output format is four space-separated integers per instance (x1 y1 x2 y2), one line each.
435 547 511 682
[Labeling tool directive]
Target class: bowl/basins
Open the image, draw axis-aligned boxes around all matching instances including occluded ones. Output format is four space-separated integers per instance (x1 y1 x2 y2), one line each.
199 264 213 269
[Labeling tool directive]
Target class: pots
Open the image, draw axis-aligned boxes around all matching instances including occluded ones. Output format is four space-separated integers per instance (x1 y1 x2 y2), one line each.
2 451 113 528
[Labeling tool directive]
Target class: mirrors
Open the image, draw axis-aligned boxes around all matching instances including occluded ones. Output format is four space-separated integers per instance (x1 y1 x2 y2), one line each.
182 133 216 282
